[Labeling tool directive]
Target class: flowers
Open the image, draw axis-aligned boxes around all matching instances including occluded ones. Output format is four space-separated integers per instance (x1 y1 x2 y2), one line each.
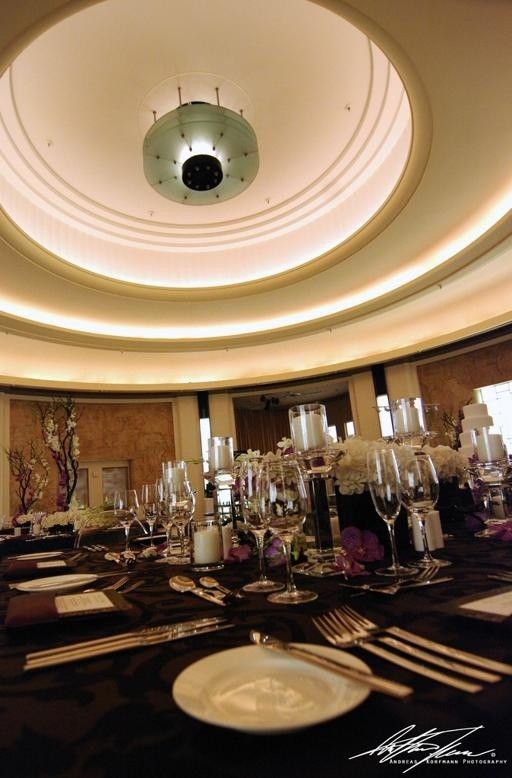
2 390 85 526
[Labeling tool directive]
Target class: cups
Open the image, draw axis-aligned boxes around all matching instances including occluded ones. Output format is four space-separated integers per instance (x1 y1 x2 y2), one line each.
208 436 235 470
286 402 327 451
190 517 232 573
470 423 506 465
14 528 22 536
391 397 427 440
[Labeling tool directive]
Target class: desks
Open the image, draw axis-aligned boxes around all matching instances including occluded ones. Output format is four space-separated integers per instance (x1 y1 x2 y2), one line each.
0 523 512 778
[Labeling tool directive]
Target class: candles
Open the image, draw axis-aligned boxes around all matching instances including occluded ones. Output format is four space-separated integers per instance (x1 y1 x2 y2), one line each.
395 407 420 433
459 430 505 464
165 444 234 486
410 510 444 551
292 412 325 450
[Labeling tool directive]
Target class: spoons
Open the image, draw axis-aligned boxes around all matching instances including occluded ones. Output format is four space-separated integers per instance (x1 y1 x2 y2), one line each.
199 576 247 599
168 574 231 607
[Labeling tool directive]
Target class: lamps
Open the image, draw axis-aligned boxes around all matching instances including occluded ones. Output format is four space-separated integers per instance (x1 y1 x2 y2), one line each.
140 83 259 205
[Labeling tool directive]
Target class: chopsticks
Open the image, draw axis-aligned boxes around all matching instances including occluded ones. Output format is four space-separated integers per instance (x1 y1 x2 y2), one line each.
8 569 135 589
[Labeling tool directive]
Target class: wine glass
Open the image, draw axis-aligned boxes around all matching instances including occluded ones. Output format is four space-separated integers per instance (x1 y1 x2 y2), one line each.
113 489 140 555
140 483 163 551
238 455 286 593
467 443 510 542
365 447 420 577
258 459 319 604
396 453 454 568
162 460 198 558
154 478 176 558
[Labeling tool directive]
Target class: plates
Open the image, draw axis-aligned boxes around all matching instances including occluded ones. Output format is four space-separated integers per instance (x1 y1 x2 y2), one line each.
15 572 101 595
169 641 373 735
13 551 65 561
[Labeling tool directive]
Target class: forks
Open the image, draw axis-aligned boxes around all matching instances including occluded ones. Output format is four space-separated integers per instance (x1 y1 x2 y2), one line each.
337 578 452 597
367 565 440 590
83 576 147 596
202 586 240 601
310 605 510 694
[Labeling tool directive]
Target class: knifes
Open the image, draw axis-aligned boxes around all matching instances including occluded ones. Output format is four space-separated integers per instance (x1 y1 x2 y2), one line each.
64 544 141 568
249 626 415 700
22 616 237 674
